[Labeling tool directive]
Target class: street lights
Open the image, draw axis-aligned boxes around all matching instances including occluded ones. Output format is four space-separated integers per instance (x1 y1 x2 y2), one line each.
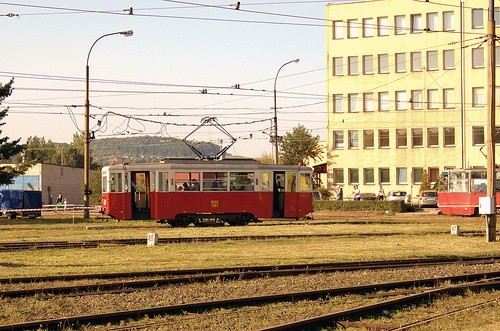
83 29 135 218
274 58 301 164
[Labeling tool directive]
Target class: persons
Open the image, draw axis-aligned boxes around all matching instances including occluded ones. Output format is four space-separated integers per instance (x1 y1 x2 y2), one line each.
56 193 63 204
175 179 253 191
337 185 385 201
274 176 285 207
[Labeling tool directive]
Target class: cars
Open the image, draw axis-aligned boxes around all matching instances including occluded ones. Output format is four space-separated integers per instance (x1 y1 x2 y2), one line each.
384 190 409 204
360 192 379 201
416 190 437 208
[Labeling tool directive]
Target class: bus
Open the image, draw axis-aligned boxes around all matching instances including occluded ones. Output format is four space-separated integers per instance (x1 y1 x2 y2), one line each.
437 129 500 218
98 116 315 229
